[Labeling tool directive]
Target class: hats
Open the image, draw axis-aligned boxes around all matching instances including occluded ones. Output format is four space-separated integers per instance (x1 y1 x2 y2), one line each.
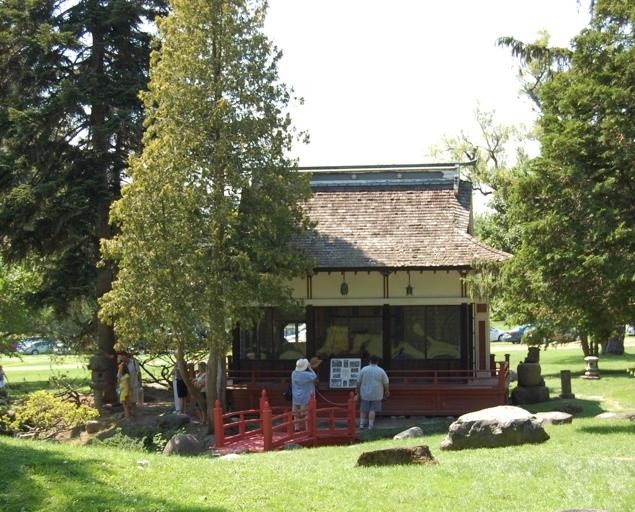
295 358 308 371
310 356 322 369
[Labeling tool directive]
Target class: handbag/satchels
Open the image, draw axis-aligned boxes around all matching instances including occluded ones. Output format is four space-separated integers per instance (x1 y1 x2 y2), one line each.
280 383 292 401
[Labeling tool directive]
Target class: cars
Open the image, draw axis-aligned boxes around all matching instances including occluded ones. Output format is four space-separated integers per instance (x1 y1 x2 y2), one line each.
18 337 71 354
490 323 578 343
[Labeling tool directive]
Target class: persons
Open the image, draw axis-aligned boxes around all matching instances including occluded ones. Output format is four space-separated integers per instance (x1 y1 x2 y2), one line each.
291 358 317 431
392 347 406 360
355 355 390 430
328 348 340 358
308 357 322 401
170 350 206 424
116 351 142 418
0 365 8 392
